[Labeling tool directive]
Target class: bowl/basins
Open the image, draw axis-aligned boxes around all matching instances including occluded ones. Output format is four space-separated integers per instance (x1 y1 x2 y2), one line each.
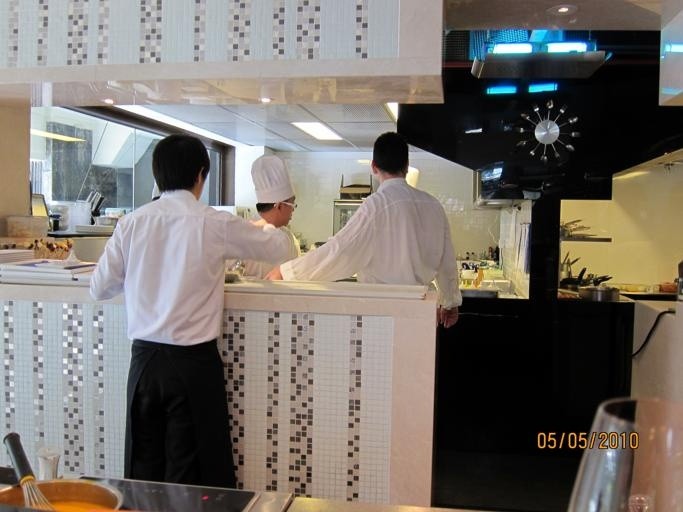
0 479 124 511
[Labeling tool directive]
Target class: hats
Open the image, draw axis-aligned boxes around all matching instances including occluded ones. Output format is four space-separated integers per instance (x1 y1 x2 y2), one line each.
250 155 294 204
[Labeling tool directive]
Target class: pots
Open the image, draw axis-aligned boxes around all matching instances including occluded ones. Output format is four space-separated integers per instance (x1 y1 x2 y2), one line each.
578 274 619 301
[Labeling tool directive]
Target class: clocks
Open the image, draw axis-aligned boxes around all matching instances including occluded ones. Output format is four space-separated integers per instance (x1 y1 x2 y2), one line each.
508 95 585 169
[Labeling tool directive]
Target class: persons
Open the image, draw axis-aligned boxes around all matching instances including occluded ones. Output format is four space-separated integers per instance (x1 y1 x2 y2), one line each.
89 135 298 490
265 131 462 327
239 154 300 278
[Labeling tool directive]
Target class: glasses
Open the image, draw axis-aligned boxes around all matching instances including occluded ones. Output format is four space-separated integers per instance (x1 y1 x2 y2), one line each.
273 201 298 212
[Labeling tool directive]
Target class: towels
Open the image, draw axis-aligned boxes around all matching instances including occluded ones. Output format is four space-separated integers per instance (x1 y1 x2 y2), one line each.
514 224 530 276
499 207 517 250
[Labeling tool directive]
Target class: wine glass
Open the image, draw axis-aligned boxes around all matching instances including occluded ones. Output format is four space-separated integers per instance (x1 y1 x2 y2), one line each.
568 399 683 512
